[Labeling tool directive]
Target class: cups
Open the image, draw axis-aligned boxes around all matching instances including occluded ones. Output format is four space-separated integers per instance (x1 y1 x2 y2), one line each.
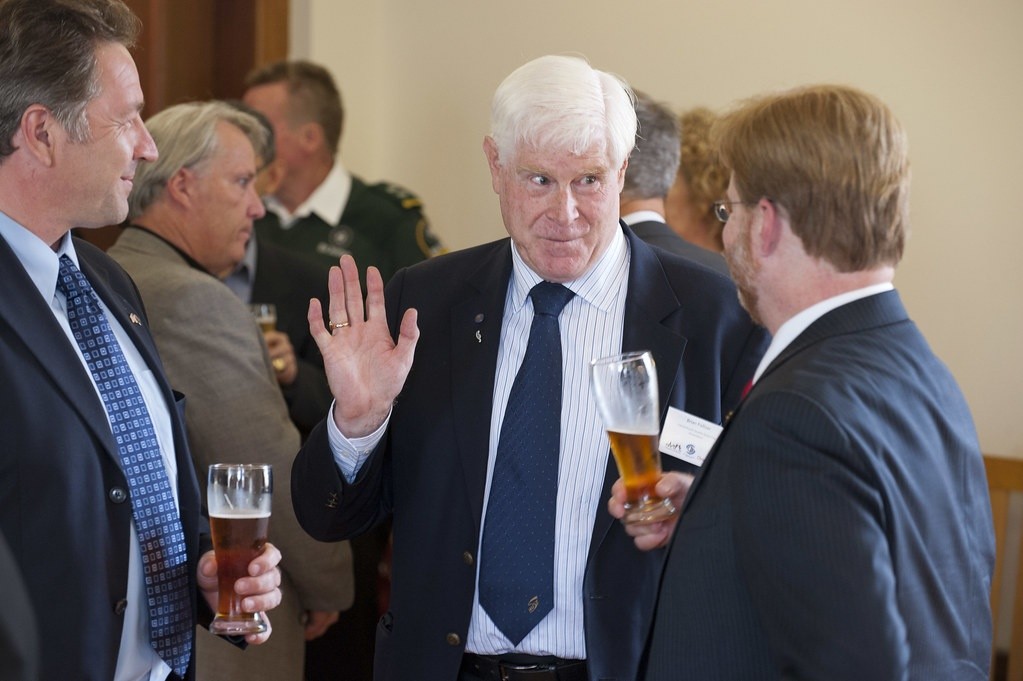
206 464 272 636
249 303 278 334
590 350 677 526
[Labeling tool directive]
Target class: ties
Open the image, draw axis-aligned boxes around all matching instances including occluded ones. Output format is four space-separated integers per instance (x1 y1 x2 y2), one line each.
56 255 193 677
479 281 576 647
741 381 751 399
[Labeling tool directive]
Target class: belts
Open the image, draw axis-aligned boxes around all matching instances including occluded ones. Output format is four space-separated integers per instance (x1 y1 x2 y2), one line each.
461 653 588 681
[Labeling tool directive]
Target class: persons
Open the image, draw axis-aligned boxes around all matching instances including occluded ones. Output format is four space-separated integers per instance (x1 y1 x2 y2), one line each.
608 86 1000 680
291 55 774 680
0 0 284 681
107 101 354 681
664 106 746 314
618 84 734 281
225 58 438 680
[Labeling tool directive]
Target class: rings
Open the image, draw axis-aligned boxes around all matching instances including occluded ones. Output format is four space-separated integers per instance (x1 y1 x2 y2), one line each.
328 321 349 330
272 358 286 371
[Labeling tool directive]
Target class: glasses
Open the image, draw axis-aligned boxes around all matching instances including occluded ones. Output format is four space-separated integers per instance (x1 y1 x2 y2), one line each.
715 200 756 222
